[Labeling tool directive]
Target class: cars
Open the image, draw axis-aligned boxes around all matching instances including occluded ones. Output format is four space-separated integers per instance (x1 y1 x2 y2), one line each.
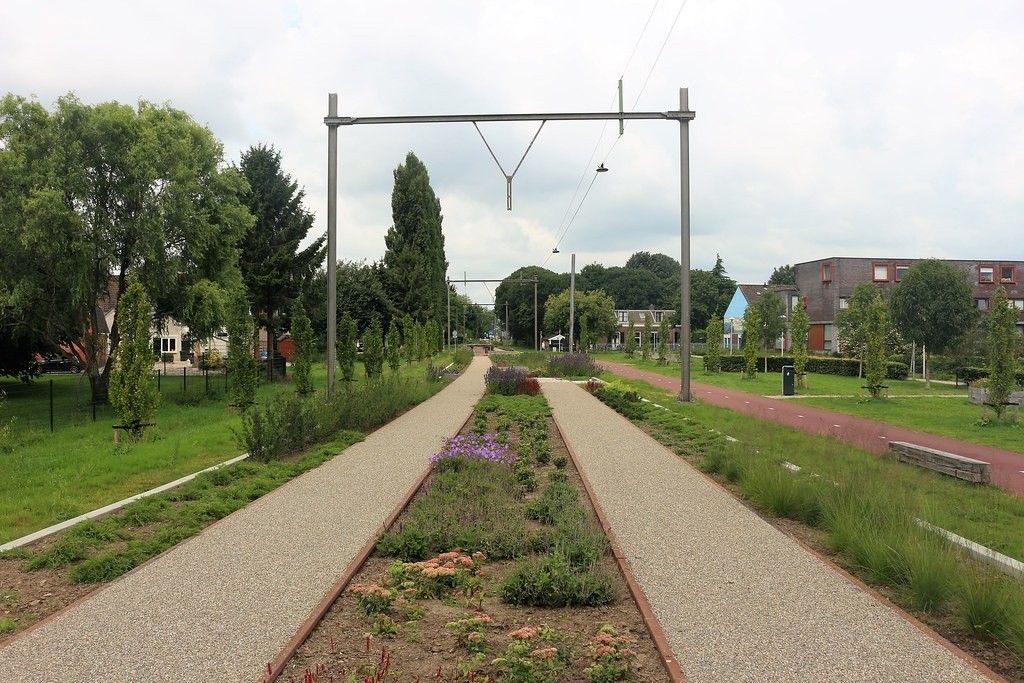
548 339 575 351
355 339 364 350
29 352 87 376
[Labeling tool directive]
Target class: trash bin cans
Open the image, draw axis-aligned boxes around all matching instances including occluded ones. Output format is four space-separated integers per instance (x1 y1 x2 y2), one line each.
782 365 794 395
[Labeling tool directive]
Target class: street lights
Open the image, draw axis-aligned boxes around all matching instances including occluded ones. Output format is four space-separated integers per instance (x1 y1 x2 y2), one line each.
779 315 787 356
729 317 735 355
